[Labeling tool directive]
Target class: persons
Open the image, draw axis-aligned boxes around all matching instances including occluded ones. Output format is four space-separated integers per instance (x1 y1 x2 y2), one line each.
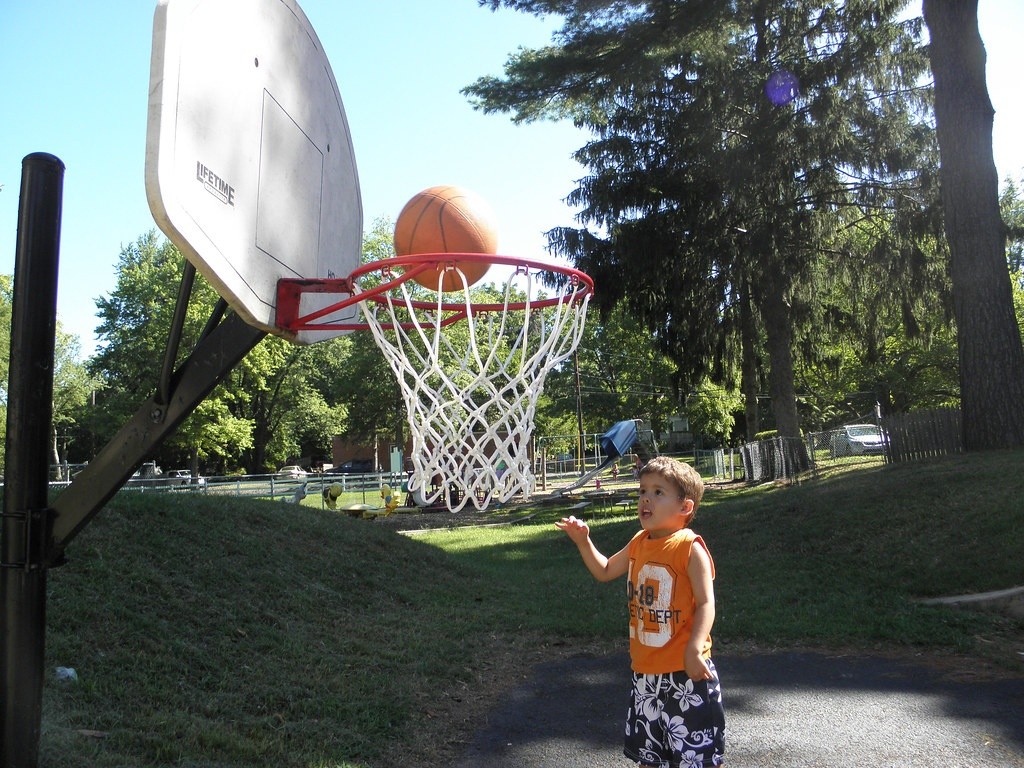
555 456 726 768
596 479 600 489
633 466 638 484
156 467 162 479
613 464 618 481
307 466 313 473
636 458 644 470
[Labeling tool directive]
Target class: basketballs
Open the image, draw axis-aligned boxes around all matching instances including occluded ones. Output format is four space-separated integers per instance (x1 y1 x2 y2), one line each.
394 187 497 292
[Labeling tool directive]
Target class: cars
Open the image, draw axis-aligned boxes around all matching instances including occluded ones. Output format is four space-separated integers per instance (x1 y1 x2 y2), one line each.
276 465 308 484
829 424 891 457
167 470 205 490
324 458 382 482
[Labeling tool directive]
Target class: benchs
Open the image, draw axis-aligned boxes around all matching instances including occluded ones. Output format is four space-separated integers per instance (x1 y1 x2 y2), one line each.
614 498 633 517
566 501 591 523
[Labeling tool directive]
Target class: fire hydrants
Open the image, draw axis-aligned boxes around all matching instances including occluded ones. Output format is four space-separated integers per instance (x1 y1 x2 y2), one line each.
611 464 620 480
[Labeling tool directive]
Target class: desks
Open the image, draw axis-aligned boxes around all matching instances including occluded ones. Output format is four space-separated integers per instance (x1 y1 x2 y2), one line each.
585 491 615 519
